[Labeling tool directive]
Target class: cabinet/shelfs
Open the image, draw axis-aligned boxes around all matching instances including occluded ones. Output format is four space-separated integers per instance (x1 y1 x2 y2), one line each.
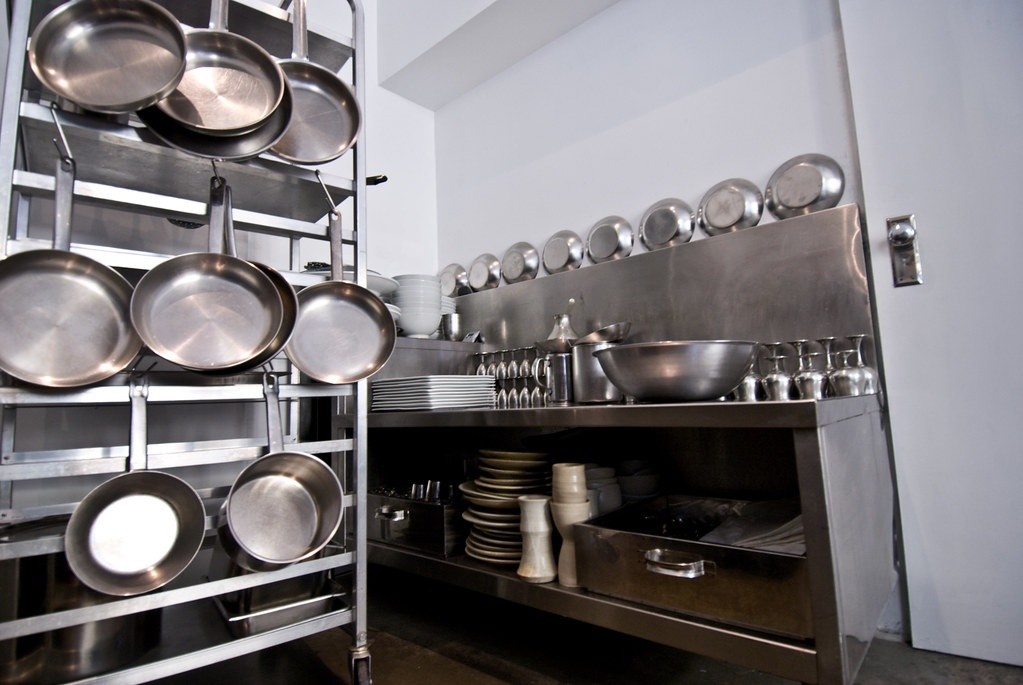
0 0 374 685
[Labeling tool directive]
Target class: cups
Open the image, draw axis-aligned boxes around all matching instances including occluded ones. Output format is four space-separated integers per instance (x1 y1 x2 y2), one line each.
442 312 465 341
533 344 621 403
518 461 621 598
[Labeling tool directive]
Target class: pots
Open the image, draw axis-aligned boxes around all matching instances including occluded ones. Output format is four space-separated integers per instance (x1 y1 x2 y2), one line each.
24 0 361 167
0 372 346 685
1 159 395 388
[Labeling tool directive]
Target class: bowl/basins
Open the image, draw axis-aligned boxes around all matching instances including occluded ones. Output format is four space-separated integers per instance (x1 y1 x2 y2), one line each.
536 338 576 356
575 321 632 345
590 340 764 402
620 476 659 496
468 154 845 293
304 265 467 340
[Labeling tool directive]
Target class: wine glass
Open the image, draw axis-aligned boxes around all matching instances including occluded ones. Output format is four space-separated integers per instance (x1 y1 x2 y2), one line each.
476 346 569 408
732 334 878 401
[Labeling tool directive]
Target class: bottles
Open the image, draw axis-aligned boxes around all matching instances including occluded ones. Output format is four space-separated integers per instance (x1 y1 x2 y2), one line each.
547 313 579 340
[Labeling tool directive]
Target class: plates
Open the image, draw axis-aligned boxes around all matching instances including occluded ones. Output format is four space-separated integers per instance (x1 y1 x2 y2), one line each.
369 375 496 413
458 444 551 566
